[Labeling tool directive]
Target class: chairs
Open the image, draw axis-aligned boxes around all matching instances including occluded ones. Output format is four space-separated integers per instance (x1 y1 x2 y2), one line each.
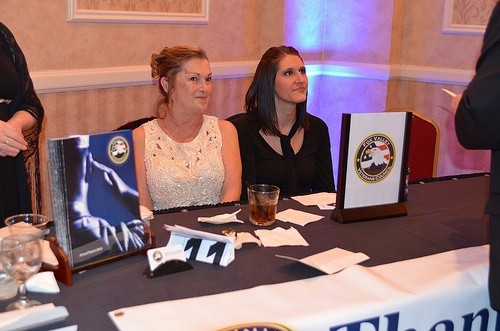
382 107 441 184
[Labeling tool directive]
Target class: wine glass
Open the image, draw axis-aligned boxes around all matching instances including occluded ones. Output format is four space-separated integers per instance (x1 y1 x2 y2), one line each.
0 234 43 311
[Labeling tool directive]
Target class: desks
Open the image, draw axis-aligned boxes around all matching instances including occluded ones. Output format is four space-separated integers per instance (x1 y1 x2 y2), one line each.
0 171 500 330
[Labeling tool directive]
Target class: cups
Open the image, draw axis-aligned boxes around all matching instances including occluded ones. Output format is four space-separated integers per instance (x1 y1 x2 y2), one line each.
247 184 280 226
4 213 50 239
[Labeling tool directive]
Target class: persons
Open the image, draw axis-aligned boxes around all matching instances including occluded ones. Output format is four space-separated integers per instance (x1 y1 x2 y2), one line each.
0 22 45 229
454 0 500 330
132 44 243 215
226 46 335 204
